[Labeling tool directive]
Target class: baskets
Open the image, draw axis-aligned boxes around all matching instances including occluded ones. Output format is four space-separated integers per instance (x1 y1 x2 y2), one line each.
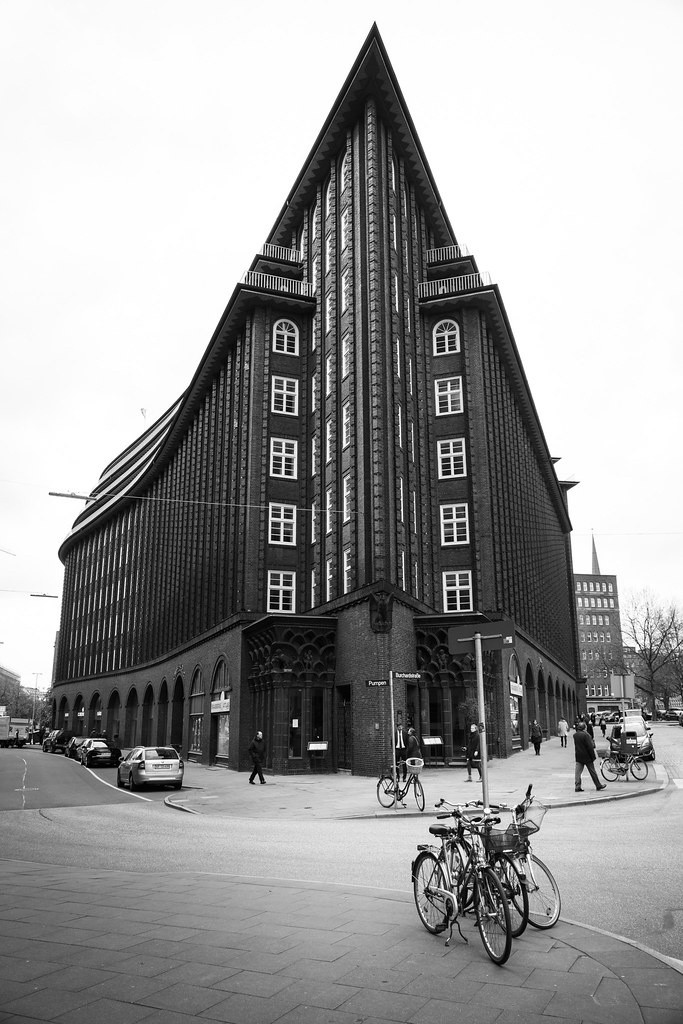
512 800 547 835
406 757 424 774
479 823 529 850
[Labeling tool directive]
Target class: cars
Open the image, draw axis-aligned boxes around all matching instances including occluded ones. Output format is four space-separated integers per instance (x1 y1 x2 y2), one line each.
80 739 122 768
63 736 108 761
607 725 656 762
585 707 683 728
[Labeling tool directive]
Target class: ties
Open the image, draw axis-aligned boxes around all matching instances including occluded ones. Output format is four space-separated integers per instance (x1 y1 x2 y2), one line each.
399 730 402 749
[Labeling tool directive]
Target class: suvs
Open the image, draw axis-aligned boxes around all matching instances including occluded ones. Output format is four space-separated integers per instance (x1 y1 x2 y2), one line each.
41 729 76 753
117 746 185 791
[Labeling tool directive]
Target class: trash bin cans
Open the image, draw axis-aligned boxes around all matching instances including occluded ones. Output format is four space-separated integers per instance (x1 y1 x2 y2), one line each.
620 730 638 754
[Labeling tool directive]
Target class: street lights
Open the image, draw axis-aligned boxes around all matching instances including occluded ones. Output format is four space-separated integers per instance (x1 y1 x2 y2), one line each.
30 672 43 744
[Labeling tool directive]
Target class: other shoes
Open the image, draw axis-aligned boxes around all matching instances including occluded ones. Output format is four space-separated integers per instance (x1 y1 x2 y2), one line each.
398 779 400 782
476 779 482 782
403 779 406 782
597 784 606 790
575 788 584 792
261 782 266 784
465 779 472 782
249 781 255 784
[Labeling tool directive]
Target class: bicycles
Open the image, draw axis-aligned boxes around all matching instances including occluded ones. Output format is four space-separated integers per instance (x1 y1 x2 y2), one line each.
376 757 426 811
600 744 649 782
409 784 563 967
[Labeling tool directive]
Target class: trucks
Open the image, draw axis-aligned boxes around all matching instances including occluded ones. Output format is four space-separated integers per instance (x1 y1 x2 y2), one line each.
0 716 31 748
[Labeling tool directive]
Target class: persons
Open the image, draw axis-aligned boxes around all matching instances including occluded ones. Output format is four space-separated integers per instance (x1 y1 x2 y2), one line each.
530 709 621 756
573 722 606 792
40 726 122 745
406 727 422 783
248 731 267 784
460 724 482 782
390 723 409 782
